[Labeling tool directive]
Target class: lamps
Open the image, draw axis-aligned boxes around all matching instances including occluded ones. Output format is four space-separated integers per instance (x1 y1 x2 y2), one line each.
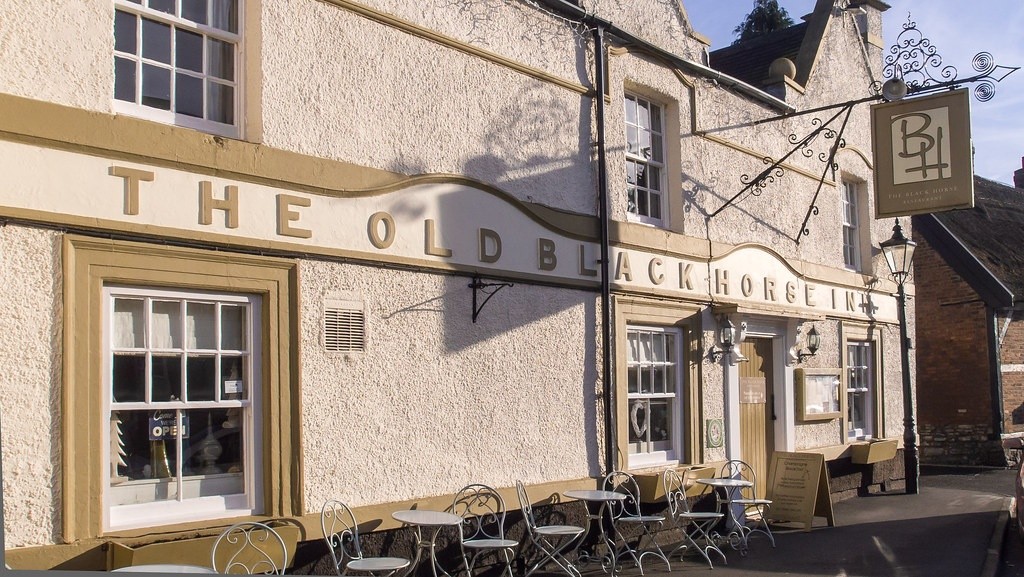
708 315 735 363
797 324 821 363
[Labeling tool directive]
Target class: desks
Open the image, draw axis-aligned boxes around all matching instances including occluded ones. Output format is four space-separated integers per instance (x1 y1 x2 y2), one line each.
694 477 754 550
563 489 626 574
390 511 462 577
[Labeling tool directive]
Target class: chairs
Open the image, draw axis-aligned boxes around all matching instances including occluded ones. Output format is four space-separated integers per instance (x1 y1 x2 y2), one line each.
212 460 775 576
